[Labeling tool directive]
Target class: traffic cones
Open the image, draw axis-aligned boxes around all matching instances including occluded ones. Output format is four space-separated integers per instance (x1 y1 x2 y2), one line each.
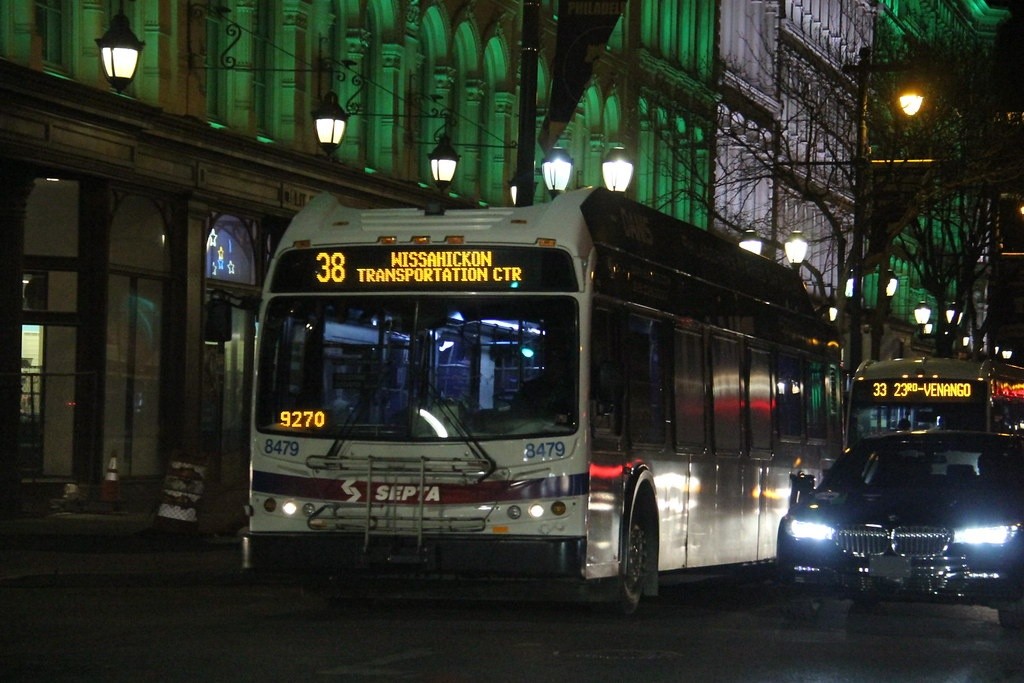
96 451 125 510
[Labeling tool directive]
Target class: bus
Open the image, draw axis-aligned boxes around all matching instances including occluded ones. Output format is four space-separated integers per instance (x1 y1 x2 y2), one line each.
841 358 1024 435
241 185 841 621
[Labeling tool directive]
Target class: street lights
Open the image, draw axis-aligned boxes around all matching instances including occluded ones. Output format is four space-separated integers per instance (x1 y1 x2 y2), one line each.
841 47 934 372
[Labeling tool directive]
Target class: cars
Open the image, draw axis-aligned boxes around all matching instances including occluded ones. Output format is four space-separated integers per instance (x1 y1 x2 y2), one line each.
771 428 1023 631
